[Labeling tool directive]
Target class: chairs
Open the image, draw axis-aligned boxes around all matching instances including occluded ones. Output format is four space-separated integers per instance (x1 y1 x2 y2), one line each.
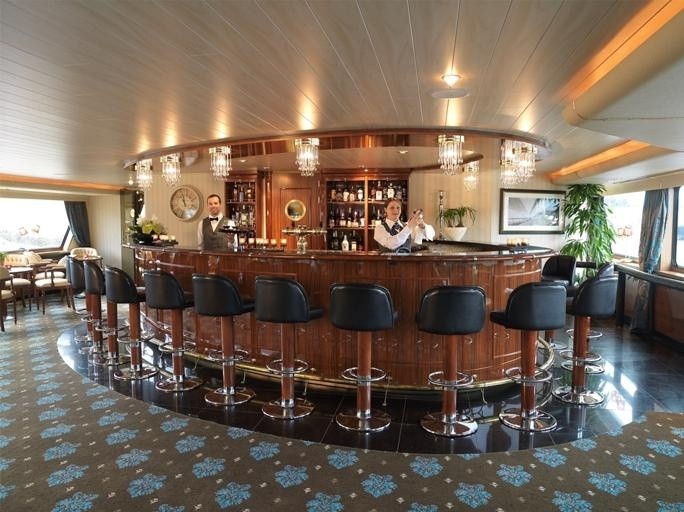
3 252 59 268
0 266 30 332
70 247 103 267
30 255 76 315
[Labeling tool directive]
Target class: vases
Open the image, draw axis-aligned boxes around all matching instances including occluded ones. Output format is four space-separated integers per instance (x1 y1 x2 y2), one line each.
143 234 154 243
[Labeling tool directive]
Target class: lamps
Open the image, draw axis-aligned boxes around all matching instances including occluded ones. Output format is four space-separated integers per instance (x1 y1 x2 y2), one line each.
295 137 320 177
208 146 232 182
160 152 183 186
499 139 537 187
463 158 480 192
437 135 466 176
136 158 153 193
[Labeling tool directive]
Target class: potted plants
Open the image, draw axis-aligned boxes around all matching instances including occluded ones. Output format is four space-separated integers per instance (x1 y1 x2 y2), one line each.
434 206 478 242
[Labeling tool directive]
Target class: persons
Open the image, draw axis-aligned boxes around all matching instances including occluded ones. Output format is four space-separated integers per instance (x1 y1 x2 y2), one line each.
197 194 238 251
373 197 436 253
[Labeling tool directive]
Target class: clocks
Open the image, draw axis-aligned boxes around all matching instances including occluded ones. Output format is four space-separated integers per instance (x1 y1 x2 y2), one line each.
168 185 205 222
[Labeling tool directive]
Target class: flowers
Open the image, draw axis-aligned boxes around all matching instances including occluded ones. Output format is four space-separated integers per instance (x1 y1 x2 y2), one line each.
136 215 166 235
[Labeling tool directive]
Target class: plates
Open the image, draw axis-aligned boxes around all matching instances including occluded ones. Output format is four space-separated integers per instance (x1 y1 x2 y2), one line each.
222 225 240 229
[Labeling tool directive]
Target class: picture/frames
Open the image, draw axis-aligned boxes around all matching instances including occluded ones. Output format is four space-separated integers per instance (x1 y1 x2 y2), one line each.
499 188 566 234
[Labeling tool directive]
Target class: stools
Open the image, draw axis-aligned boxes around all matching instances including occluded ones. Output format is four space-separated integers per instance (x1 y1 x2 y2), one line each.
192 273 255 405
255 276 325 418
537 254 577 350
490 281 566 432
414 285 486 437
105 266 160 380
329 283 399 432
552 262 619 405
144 270 206 392
66 256 108 355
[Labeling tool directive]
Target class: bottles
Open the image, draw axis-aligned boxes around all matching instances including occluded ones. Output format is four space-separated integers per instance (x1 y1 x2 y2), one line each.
231 205 256 229
367 180 406 202
331 229 363 252
329 205 364 227
330 181 363 202
233 230 256 252
371 205 403 228
233 181 255 202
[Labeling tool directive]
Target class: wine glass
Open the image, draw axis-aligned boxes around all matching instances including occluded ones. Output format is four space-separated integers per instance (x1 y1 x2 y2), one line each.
239 236 287 254
506 235 529 255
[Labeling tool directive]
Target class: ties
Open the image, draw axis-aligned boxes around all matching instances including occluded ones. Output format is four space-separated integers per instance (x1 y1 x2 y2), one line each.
394 221 399 232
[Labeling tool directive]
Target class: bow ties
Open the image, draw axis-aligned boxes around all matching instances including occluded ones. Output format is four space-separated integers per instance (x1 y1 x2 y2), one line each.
209 217 219 222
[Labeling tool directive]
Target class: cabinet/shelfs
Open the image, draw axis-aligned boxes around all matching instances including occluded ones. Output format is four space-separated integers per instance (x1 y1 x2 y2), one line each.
225 179 258 235
322 176 410 250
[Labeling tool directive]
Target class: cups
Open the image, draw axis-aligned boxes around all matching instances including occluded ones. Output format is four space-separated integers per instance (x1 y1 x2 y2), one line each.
152 234 176 242
